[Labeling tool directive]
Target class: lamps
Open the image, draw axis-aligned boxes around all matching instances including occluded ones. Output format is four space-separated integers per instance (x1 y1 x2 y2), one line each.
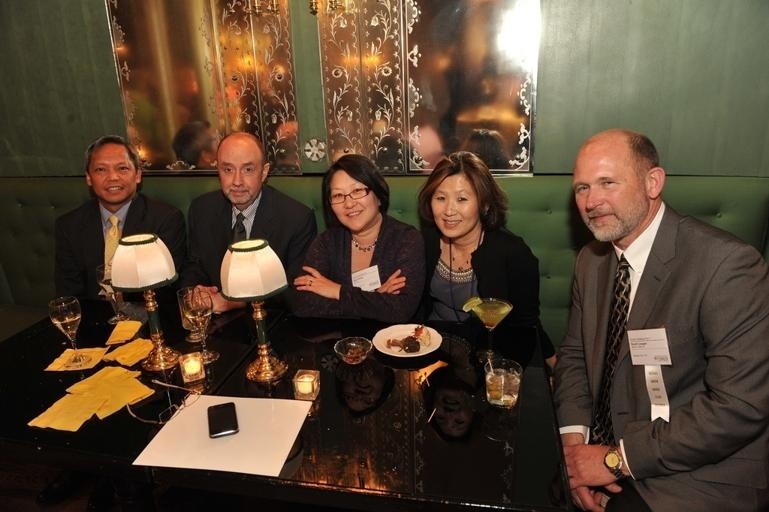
219 239 289 383
111 232 182 372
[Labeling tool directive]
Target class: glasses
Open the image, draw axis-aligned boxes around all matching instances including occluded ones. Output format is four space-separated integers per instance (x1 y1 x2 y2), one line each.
329 188 373 206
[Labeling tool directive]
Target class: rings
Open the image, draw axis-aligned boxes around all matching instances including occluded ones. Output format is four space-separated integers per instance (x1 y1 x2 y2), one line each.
308 280 312 286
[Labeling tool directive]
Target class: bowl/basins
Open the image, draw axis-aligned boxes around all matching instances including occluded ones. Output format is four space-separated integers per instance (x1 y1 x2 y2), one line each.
333 336 373 366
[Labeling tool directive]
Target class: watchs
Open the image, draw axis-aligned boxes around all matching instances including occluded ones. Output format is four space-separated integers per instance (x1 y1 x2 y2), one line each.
605 442 634 485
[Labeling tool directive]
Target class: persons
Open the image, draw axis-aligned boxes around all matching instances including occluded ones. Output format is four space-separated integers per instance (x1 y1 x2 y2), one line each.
410 321 538 446
409 151 557 371
171 120 222 170
269 119 302 174
177 133 317 316
462 129 511 174
551 128 769 512
291 315 396 418
284 154 426 323
52 135 187 307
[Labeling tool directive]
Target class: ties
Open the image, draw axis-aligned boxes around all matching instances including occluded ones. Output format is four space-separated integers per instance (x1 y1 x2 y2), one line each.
230 215 245 245
102 214 122 282
587 255 632 449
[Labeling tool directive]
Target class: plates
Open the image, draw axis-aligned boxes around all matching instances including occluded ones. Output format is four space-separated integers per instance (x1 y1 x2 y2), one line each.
371 323 443 359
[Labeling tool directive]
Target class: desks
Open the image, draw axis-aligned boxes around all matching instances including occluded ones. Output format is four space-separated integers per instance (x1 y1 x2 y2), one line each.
0 312 575 512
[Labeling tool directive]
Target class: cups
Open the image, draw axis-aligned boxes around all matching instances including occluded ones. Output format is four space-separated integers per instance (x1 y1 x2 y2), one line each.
483 356 524 410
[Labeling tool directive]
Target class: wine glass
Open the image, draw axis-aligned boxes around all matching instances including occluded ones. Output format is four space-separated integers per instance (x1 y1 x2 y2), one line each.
94 263 131 325
49 294 92 369
182 290 220 365
470 297 514 364
176 287 209 343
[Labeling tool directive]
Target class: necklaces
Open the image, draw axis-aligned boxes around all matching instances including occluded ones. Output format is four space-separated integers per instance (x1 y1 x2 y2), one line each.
351 236 378 252
449 249 472 271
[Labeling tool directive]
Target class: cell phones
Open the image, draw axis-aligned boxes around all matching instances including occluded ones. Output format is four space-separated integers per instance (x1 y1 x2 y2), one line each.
207 402 239 438
